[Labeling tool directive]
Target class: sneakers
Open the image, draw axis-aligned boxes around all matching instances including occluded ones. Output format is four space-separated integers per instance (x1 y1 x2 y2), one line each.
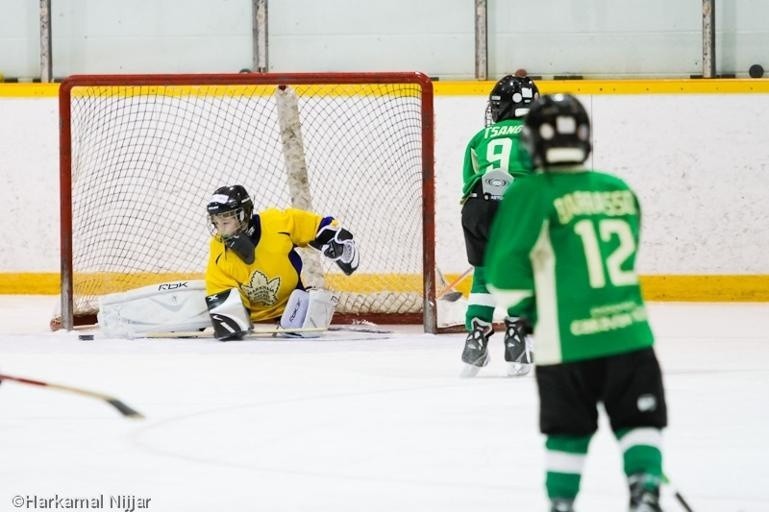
552 499 573 512
504 316 533 364
460 316 494 366
628 489 662 512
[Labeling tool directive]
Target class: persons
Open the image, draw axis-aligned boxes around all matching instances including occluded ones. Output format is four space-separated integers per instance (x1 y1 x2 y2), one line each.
200 184 358 341
484 93 670 511
462 73 546 372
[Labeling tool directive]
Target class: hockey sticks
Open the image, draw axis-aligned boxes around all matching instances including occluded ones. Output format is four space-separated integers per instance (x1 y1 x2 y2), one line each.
434 266 474 302
78 324 391 340
1 375 144 419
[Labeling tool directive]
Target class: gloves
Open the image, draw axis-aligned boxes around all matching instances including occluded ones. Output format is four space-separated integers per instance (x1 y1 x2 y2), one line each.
315 224 360 275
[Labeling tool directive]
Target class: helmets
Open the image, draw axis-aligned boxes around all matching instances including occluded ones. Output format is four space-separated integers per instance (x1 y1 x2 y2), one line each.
517 91 591 171
484 74 540 127
206 185 254 243
